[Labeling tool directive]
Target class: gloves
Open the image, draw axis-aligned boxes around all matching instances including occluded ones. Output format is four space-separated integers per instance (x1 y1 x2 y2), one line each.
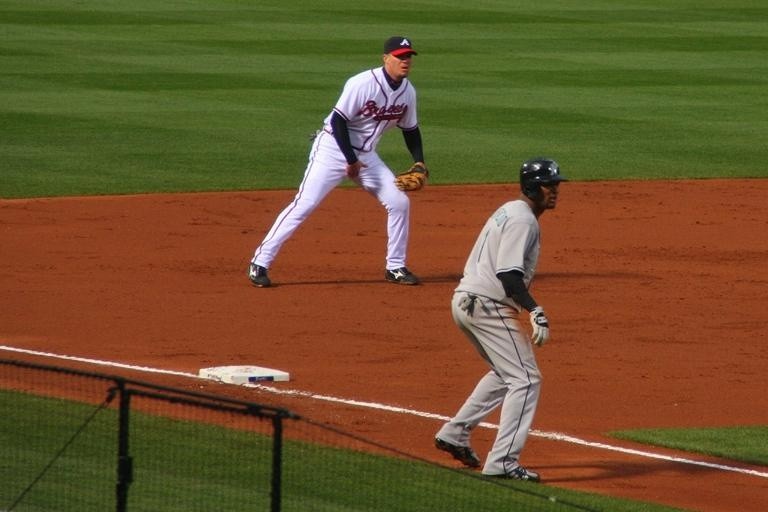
528 305 550 345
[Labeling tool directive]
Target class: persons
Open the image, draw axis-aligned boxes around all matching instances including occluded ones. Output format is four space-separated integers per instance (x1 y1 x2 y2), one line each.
247 36 430 287
434 156 570 482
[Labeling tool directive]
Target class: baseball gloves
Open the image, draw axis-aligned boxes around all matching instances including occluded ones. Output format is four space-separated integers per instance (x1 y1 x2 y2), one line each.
394 162 427 192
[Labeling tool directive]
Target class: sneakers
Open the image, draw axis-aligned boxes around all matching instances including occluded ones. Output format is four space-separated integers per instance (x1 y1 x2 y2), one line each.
385 265 419 286
246 262 272 288
434 436 482 468
494 464 539 484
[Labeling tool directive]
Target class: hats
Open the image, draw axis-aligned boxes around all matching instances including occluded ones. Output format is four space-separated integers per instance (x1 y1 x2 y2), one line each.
383 35 418 58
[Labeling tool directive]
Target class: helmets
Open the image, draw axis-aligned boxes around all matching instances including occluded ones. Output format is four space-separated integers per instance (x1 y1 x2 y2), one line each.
518 157 569 203
